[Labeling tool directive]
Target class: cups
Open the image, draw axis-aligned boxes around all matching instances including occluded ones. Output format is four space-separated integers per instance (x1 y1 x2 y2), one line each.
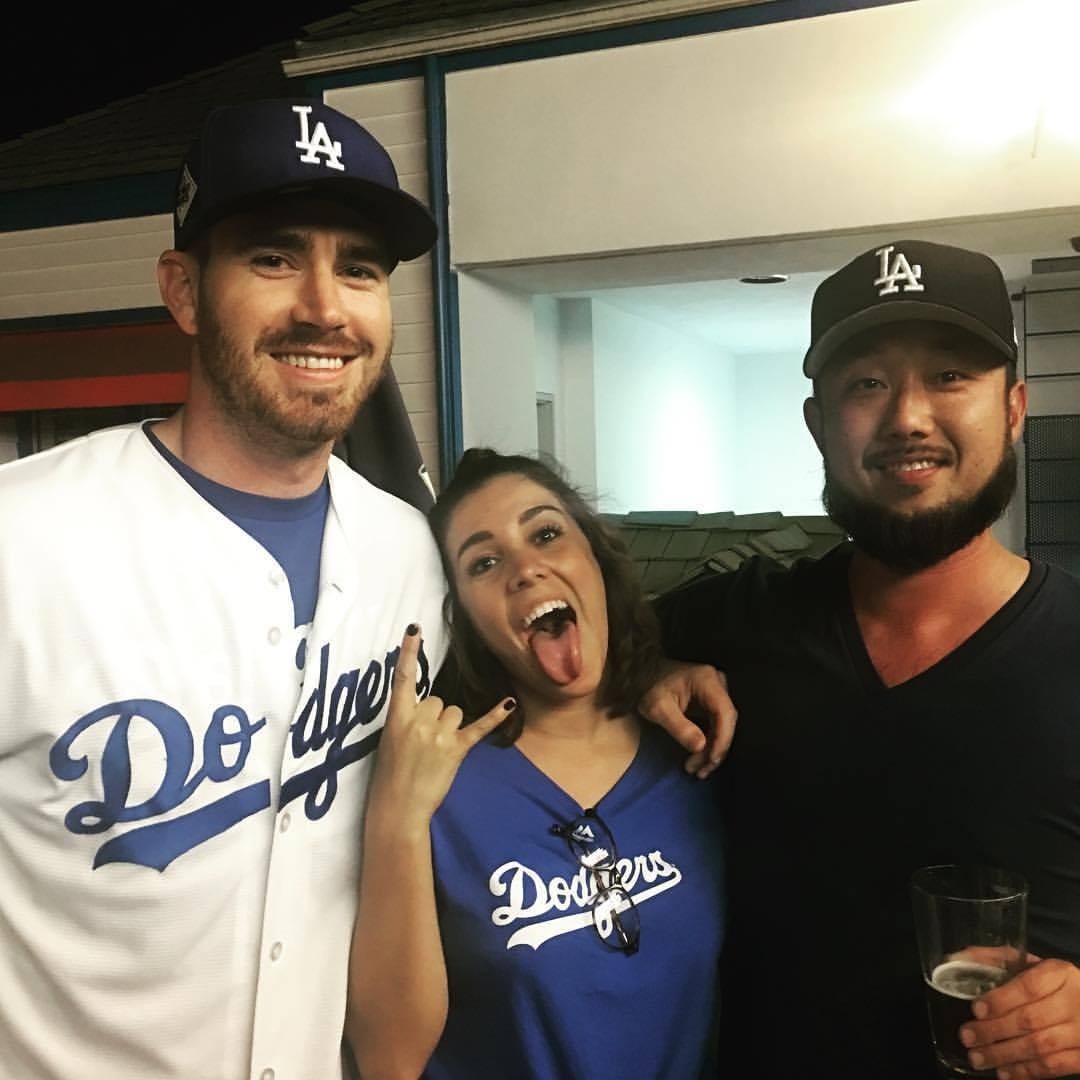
905 864 1030 1079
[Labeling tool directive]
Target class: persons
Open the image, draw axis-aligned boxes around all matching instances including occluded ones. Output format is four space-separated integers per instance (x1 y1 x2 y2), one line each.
430 239 1080 1080
0 95 740 1080
342 446 734 1080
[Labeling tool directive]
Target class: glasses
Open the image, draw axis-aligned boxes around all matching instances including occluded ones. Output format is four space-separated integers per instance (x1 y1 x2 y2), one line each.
550 808 641 957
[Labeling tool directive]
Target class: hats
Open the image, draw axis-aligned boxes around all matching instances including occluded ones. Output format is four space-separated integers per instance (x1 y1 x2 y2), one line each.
803 240 1017 377
174 97 439 277
